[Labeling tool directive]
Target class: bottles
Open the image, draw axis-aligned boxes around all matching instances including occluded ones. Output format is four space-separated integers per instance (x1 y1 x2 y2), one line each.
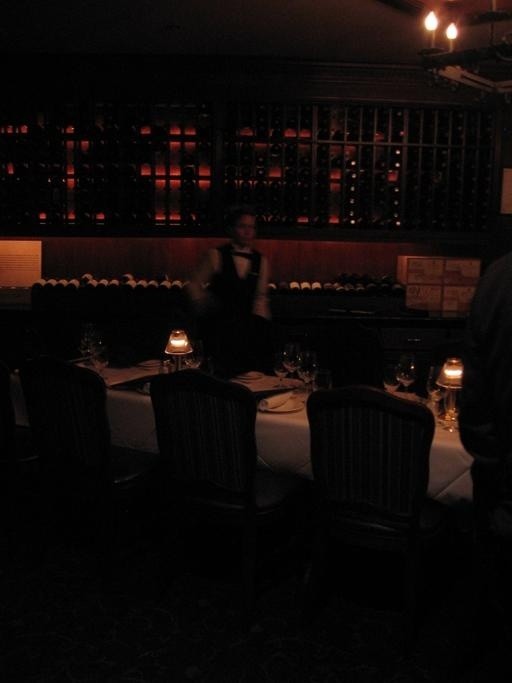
265 278 363 291
29 270 181 291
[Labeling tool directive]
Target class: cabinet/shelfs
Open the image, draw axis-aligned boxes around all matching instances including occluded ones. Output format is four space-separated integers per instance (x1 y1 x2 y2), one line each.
1 92 502 244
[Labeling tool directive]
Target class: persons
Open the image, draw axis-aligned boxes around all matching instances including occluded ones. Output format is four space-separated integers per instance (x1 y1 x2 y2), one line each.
191 207 270 318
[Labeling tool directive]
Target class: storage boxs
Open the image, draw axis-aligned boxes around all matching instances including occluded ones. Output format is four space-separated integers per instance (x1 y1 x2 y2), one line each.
395 254 482 313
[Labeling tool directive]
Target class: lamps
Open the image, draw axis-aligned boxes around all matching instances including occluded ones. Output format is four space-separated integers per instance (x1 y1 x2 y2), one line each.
164 328 193 372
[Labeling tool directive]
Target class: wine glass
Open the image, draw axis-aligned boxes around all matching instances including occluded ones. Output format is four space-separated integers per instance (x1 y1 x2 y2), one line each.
384 360 416 399
275 349 318 397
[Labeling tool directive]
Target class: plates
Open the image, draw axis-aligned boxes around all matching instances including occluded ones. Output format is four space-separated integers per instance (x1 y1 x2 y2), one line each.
257 398 305 413
236 374 265 380
139 362 161 367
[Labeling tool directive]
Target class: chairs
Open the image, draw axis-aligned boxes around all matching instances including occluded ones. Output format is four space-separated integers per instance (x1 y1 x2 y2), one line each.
149 372 258 634
297 384 436 639
17 361 147 595
